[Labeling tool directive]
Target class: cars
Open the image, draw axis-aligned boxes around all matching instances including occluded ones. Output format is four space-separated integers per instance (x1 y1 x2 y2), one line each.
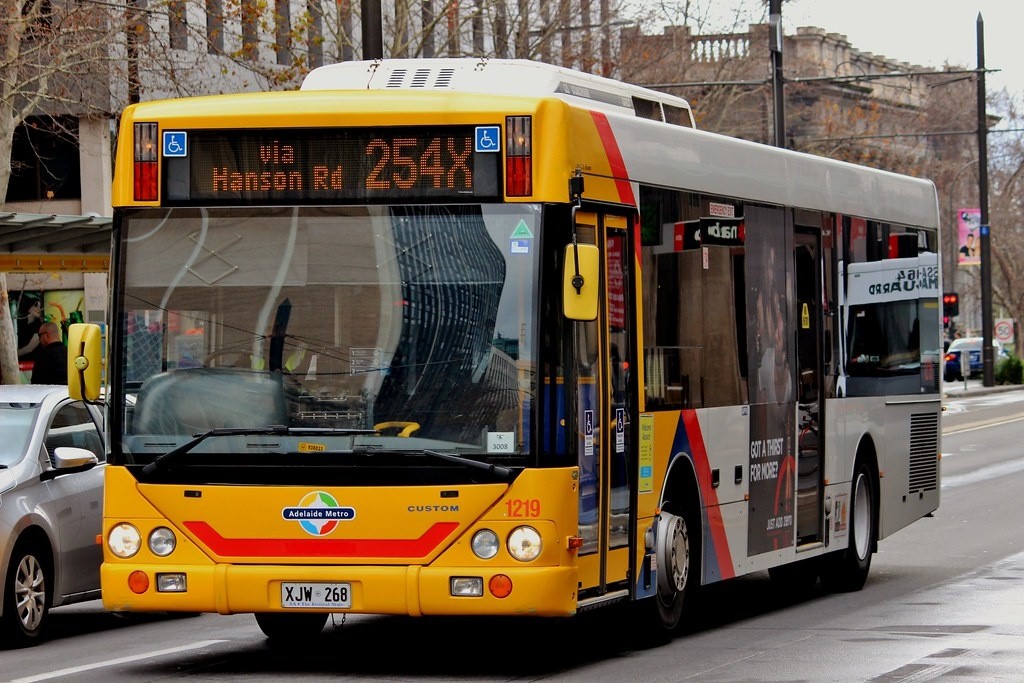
0 385 146 641
943 337 1012 383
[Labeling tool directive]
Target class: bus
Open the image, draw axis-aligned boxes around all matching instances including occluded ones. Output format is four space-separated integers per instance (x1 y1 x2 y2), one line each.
68 93 944 650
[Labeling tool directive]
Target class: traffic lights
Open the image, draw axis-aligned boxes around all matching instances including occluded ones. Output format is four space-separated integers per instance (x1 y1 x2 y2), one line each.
943 292 958 316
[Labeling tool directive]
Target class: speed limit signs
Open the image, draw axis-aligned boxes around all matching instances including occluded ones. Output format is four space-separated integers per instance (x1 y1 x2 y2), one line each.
994 322 1013 339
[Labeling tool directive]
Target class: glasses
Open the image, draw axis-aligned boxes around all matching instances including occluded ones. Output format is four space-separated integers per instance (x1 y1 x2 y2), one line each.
38 332 47 337
34 304 42 309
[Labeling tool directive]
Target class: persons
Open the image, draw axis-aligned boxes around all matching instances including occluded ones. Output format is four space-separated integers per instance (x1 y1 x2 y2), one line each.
754 230 795 451
30 322 69 385
18 296 45 362
231 305 318 393
954 332 962 338
960 234 980 258
